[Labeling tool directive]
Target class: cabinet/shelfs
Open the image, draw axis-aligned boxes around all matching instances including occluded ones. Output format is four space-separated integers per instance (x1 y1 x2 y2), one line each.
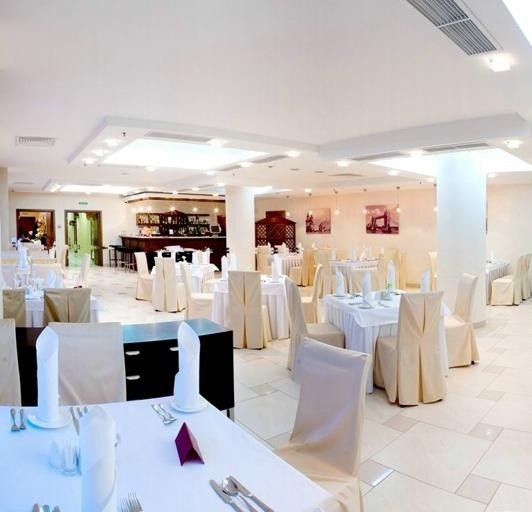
136 212 212 236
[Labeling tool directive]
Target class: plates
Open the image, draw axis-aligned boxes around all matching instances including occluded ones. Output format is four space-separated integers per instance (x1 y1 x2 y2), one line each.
219 278 227 282
25 285 44 300
329 288 397 311
327 255 378 263
169 395 208 413
269 274 285 284
27 409 72 429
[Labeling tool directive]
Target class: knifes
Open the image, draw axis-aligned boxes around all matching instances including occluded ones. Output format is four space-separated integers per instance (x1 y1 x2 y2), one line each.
69 406 80 435
208 475 276 512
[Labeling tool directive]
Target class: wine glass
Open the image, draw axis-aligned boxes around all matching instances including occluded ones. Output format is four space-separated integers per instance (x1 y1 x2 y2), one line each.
72 272 81 288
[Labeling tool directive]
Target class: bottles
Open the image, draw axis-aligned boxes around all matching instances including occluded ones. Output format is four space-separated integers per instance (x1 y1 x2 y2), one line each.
161 216 210 236
16 246 33 284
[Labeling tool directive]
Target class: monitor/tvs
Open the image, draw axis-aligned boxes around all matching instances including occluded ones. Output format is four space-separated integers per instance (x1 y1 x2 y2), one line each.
209 225 222 237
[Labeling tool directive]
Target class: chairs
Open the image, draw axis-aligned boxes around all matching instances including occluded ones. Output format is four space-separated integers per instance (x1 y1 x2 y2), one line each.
1 238 96 322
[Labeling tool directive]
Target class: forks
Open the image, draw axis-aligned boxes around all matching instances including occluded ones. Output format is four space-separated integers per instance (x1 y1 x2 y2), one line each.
120 491 144 512
151 402 177 426
9 407 26 433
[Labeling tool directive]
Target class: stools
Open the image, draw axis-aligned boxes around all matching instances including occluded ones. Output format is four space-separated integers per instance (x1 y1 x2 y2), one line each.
109 244 139 274
